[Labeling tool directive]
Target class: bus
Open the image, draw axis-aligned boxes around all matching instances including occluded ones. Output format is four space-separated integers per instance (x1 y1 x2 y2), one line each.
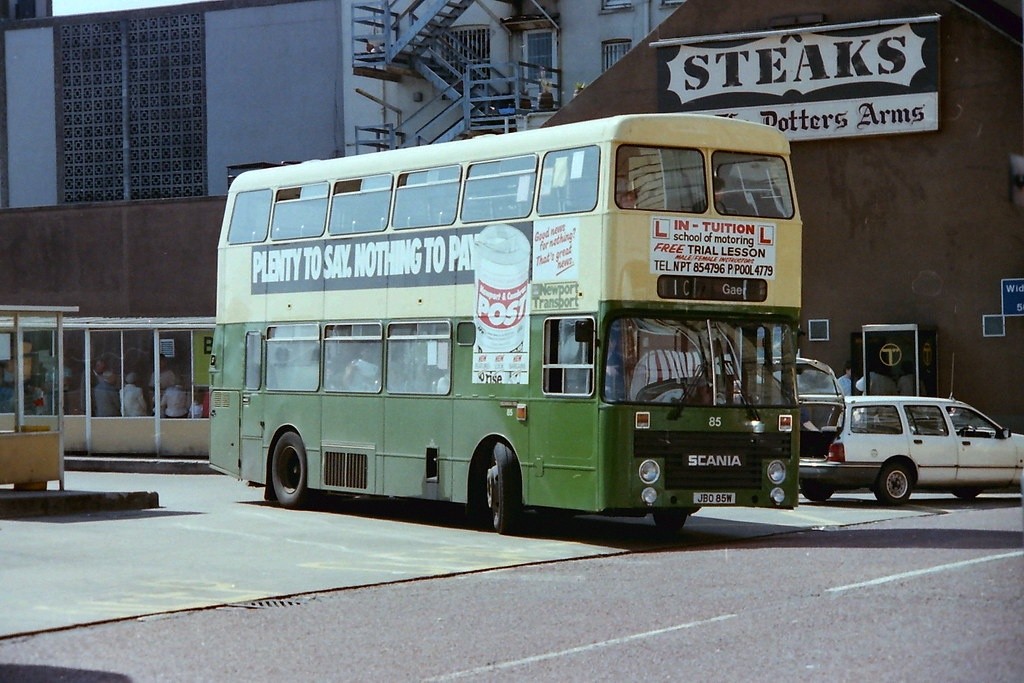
206 111 808 535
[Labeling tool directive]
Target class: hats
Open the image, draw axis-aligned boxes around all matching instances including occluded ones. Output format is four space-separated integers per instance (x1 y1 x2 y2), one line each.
124 372 136 383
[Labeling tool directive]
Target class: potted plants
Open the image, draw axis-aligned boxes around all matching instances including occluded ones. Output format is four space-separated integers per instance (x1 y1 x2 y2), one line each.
520 91 531 109
573 82 585 97
538 80 553 109
498 93 515 115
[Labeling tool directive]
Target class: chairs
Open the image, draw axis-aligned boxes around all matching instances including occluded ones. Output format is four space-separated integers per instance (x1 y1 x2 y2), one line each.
885 415 901 430
253 203 528 242
922 412 945 431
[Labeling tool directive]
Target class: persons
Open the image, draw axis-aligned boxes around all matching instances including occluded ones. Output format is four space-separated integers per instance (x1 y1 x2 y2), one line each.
838 361 926 396
692 177 727 214
80 355 208 418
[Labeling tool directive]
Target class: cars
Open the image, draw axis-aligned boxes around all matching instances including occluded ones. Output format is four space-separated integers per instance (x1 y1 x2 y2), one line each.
5 354 88 415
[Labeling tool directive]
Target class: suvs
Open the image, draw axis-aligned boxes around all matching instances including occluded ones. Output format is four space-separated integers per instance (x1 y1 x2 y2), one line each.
757 358 1023 506
